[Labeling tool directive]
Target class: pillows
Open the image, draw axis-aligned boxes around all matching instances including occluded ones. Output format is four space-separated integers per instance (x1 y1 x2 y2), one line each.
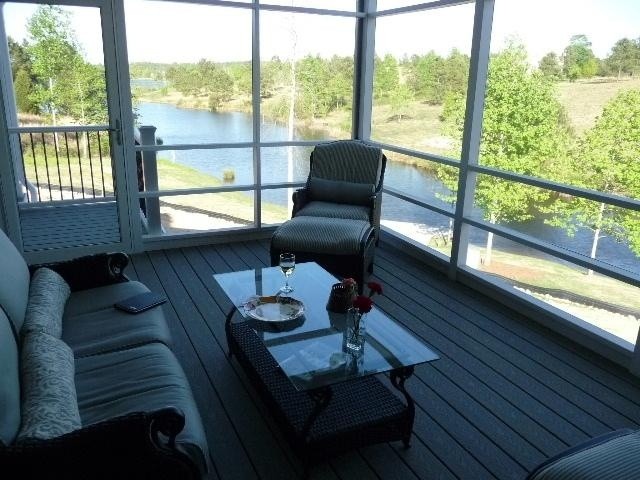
16 266 83 443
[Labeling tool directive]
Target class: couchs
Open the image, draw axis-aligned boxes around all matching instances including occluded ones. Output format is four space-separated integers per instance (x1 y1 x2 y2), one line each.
0 229 211 479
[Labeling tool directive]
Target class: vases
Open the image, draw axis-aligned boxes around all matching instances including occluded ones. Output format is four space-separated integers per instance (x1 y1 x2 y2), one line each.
342 307 368 358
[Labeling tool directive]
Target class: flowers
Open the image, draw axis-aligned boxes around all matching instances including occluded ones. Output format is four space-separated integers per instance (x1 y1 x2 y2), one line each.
340 279 384 343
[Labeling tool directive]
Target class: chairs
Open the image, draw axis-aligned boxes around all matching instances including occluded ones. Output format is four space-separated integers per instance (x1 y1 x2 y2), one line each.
271 140 388 296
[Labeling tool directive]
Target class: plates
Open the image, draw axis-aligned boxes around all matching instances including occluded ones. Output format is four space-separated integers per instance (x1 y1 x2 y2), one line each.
243 296 305 324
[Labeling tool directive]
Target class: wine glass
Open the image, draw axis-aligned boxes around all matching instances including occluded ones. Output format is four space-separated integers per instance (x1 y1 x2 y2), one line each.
279 253 296 294
